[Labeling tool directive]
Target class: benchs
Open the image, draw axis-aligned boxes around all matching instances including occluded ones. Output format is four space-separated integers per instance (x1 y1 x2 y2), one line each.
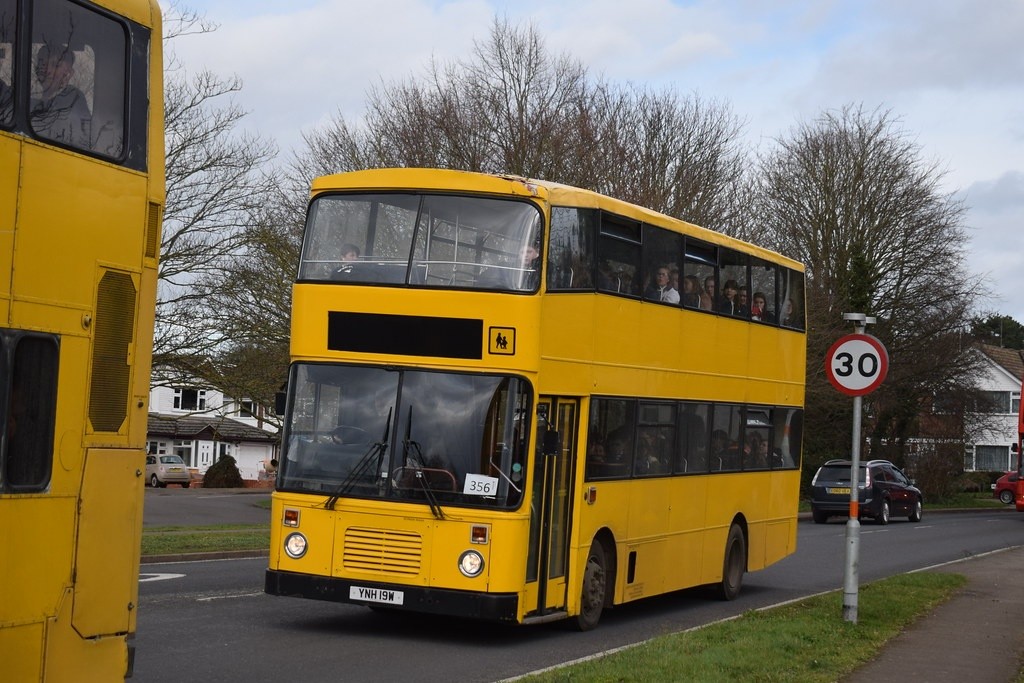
585 446 788 478
316 249 781 326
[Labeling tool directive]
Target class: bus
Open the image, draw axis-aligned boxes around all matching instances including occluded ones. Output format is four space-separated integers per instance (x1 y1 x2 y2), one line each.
260 169 814 637
1 1 166 681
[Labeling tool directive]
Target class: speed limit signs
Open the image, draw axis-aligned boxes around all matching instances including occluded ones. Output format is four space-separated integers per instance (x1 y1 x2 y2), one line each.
825 334 890 395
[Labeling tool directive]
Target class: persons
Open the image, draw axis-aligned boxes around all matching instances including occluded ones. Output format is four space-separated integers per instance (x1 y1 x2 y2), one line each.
684 274 795 326
654 265 681 305
508 240 550 290
589 424 785 469
329 243 361 281
29 43 96 152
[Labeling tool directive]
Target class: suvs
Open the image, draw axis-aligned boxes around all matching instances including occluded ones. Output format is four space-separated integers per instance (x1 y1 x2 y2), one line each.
813 458 926 522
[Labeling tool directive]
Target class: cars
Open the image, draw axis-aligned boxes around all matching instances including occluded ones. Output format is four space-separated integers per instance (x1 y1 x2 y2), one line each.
145 451 192 489
992 470 1020 504
